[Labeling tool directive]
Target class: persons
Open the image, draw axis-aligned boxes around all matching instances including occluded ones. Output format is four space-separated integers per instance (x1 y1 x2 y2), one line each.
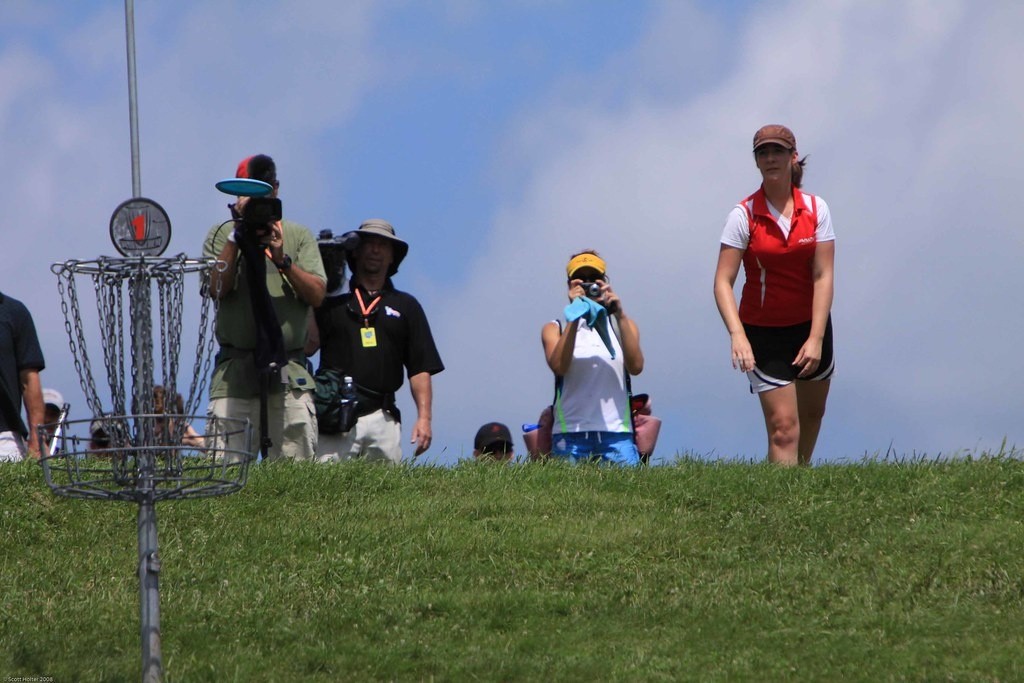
472 421 514 465
42 390 68 455
0 292 51 462
132 385 206 464
199 153 446 468
714 125 836 468
541 250 644 470
89 412 131 459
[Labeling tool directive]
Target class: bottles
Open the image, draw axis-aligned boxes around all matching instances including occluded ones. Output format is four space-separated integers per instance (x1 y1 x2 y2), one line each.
179 445 205 458
341 377 357 430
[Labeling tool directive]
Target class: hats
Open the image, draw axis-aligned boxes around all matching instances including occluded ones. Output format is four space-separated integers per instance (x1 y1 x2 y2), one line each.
90 415 125 439
752 124 796 152
566 254 606 277
42 388 64 411
235 155 276 181
341 219 408 276
474 422 514 451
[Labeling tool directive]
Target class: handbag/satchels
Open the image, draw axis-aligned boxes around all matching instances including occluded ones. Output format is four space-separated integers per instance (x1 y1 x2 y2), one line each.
310 364 382 436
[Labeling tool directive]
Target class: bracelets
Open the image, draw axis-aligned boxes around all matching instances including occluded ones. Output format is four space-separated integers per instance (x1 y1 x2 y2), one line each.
228 228 239 242
277 253 291 270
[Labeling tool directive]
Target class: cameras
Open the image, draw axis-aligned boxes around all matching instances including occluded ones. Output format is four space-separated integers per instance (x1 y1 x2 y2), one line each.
581 282 601 296
